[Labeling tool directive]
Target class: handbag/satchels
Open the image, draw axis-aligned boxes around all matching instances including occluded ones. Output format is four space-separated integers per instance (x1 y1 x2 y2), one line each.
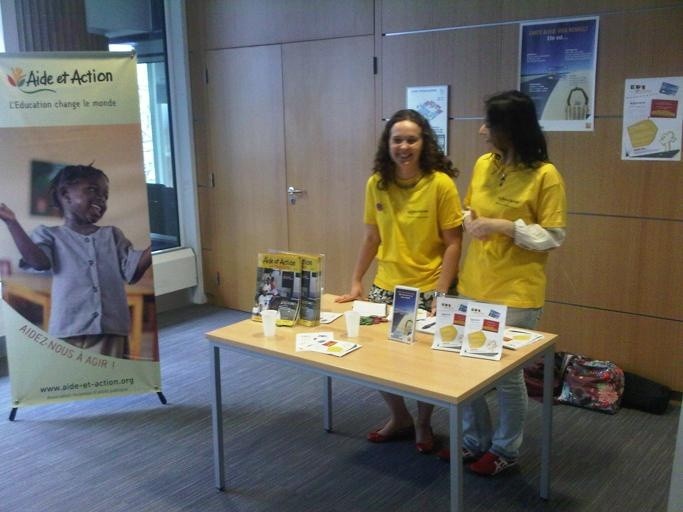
523 351 672 415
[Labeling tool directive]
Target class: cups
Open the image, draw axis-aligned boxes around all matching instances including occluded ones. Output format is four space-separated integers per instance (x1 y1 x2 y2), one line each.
260 310 279 337
345 310 360 337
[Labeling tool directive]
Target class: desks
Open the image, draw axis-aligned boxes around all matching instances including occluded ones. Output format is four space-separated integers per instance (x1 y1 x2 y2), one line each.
204 293 559 512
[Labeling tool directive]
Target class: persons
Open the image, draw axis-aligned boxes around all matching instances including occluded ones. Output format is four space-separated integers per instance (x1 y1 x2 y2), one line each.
0 161 152 358
335 110 465 452
456 90 568 475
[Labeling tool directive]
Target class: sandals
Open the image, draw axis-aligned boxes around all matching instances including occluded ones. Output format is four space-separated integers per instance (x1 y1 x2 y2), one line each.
416 427 435 453
367 425 415 442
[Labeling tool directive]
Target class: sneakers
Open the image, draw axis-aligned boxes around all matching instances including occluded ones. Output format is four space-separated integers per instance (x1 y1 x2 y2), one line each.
435 448 519 476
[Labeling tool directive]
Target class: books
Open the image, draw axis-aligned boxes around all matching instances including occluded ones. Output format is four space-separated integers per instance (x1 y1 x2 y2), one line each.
251 250 325 327
